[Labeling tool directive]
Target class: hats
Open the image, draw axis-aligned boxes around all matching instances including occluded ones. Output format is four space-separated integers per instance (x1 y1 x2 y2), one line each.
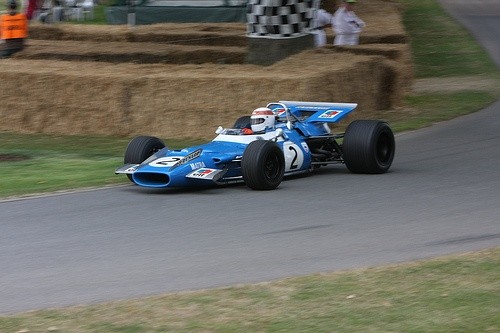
344 0 356 3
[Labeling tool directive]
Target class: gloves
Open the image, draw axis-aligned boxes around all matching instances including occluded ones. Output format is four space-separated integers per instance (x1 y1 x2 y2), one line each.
242 127 253 134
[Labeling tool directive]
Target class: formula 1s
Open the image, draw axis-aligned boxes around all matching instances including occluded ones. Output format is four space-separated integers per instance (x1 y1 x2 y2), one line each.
115 98 397 191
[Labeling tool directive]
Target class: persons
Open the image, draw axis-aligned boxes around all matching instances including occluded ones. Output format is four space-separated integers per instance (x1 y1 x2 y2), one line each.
0 0 28 54
314 3 335 46
46 1 65 23
331 0 366 46
240 106 277 136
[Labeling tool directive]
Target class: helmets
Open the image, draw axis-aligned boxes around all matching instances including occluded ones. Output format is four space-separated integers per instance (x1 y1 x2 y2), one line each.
250 106 274 130
